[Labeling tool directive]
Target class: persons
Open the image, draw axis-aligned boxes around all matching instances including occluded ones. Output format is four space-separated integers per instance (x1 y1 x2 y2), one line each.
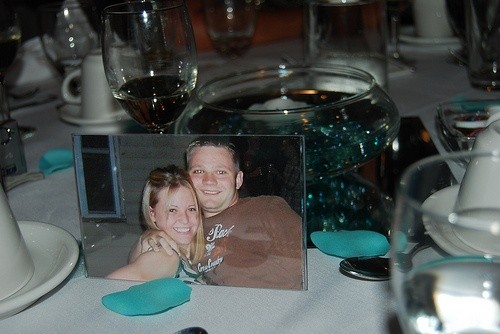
103 166 207 285
128 137 302 290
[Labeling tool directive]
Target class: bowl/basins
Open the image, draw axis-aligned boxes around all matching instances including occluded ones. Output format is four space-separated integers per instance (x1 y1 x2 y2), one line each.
397 24 464 65
172 65 401 183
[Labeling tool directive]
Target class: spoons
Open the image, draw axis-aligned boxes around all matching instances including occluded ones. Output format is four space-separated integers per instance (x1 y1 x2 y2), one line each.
339 234 435 281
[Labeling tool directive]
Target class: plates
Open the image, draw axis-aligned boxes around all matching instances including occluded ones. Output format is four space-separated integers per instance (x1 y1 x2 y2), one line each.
422 184 495 256
0 221 79 320
58 105 140 133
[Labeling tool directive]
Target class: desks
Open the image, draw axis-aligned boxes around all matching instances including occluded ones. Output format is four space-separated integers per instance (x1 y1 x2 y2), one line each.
0 36 500 334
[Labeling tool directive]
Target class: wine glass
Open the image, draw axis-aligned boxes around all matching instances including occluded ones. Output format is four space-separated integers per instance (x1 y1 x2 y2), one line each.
101 0 198 134
200 0 256 74
0 0 37 140
40 4 99 111
436 99 500 152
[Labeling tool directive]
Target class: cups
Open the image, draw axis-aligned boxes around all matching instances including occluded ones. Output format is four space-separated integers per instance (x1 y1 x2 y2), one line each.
60 49 126 119
388 151 500 334
465 0 500 92
302 0 389 98
0 119 28 177
412 0 455 37
0 181 35 301
450 118 500 256
